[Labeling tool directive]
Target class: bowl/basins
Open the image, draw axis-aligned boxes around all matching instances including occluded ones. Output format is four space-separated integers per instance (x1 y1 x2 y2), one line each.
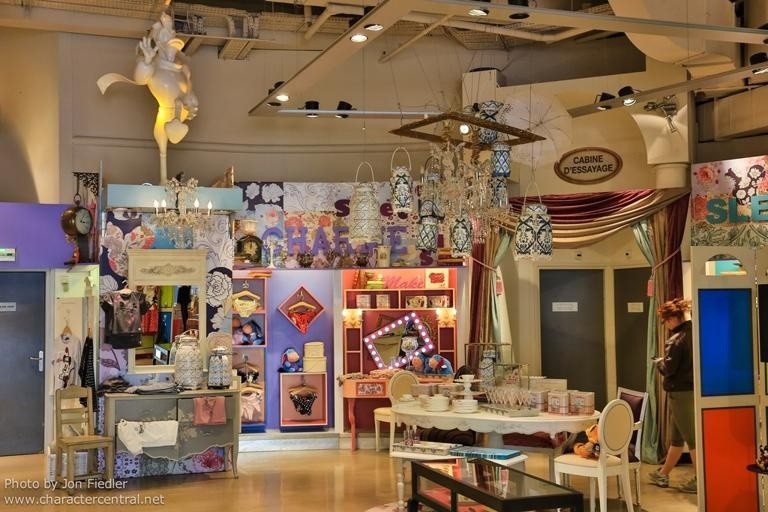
424 393 449 412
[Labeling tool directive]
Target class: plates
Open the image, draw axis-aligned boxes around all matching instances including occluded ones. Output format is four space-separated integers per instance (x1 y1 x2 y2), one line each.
400 398 416 401
452 399 478 414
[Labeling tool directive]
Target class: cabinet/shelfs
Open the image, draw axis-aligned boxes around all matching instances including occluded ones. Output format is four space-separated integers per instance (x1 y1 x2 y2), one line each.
338 267 458 436
43 264 98 484
102 205 234 388
231 269 272 431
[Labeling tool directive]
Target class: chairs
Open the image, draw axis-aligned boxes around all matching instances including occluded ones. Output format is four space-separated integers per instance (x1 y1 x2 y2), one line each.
388 374 464 506
554 400 634 512
373 371 418 450
54 386 111 495
616 387 648 505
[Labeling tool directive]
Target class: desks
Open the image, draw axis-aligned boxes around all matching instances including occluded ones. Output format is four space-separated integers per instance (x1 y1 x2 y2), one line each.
341 372 448 451
413 454 584 511
105 391 239 484
392 400 601 450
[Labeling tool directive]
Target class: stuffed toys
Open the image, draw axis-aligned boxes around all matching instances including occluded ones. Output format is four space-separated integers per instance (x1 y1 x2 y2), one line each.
413 353 431 375
282 348 302 373
429 355 453 374
574 424 600 457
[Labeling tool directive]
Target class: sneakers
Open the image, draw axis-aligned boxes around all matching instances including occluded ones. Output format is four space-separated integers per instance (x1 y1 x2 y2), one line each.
647 468 670 487
678 475 698 493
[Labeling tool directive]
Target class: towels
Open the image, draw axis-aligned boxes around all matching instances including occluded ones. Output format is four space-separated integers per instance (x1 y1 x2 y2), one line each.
193 397 227 426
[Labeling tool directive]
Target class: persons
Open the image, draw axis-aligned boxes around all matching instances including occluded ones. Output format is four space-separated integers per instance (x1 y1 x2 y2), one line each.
647 299 698 492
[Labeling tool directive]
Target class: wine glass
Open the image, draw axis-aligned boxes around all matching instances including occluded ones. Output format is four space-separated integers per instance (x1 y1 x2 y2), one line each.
484 386 536 417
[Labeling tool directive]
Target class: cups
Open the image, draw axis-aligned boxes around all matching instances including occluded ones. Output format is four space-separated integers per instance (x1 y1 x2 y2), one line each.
459 374 475 380
403 394 412 400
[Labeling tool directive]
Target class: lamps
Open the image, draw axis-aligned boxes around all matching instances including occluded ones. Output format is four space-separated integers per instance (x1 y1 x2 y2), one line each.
346 103 556 263
267 89 281 107
617 85 637 105
594 91 615 111
335 101 352 119
459 125 470 135
349 17 369 44
273 81 290 102
750 53 768 74
363 5 383 32
304 101 318 118
468 1 490 17
